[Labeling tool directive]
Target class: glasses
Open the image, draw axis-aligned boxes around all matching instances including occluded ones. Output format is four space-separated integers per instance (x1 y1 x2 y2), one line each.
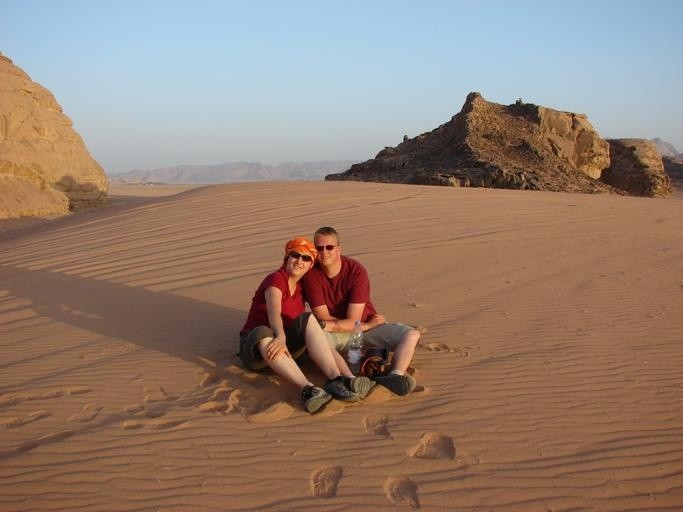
315 245 335 252
289 250 312 262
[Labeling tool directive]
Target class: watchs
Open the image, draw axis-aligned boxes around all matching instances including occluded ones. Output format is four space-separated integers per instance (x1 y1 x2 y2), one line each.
320 320 327 329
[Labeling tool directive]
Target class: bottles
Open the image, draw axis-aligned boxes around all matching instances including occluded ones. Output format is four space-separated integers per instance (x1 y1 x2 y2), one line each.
346 320 364 374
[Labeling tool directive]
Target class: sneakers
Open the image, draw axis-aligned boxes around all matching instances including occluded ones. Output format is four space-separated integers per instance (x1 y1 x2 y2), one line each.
323 376 376 402
302 385 332 414
376 370 416 396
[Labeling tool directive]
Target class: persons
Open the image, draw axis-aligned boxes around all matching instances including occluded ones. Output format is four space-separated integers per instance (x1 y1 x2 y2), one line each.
301 226 421 396
239 237 360 414
